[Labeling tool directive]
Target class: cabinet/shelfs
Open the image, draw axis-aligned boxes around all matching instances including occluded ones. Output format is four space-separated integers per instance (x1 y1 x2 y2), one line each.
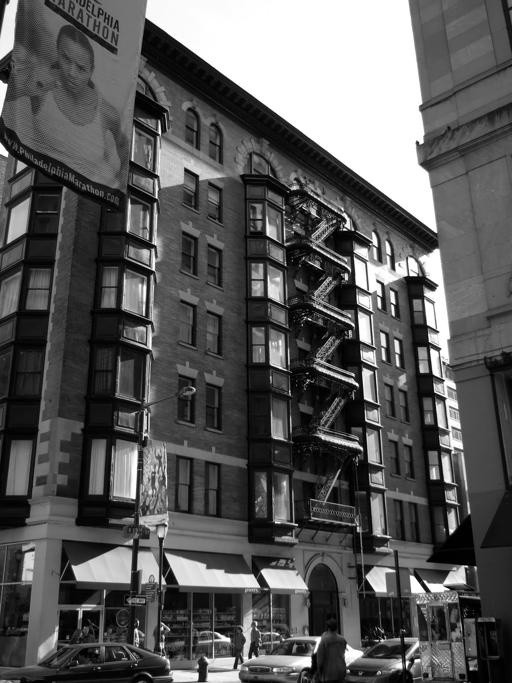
163 608 288 656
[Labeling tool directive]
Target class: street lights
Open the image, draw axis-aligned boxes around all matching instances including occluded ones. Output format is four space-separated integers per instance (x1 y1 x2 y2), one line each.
150 520 169 654
129 387 198 648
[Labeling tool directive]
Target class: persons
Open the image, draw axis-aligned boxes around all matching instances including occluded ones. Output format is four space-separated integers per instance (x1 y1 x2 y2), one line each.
1 22 131 193
134 618 143 648
316 618 348 683
248 621 262 660
153 622 170 657
232 625 246 670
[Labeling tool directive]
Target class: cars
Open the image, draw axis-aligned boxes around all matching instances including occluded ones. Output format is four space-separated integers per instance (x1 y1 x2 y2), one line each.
238 636 362 683
0 642 174 683
346 636 424 682
166 628 232 657
258 629 284 652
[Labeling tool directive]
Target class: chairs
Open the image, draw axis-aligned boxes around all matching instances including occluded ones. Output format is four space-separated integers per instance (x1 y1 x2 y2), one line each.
114 651 128 661
